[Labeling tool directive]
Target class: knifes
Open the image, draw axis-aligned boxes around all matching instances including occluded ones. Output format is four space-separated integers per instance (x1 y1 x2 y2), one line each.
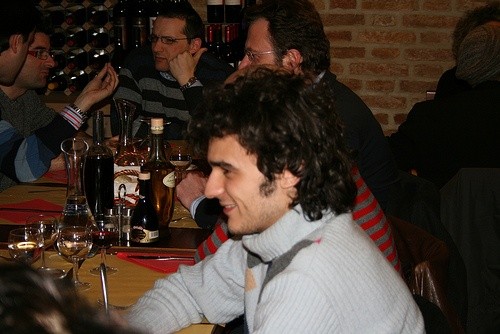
127 255 194 261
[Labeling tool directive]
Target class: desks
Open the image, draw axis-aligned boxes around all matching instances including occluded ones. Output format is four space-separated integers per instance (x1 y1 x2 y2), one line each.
0 170 216 334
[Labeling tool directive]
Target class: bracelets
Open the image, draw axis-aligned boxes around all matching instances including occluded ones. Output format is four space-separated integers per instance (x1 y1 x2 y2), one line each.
179 76 198 92
69 103 87 119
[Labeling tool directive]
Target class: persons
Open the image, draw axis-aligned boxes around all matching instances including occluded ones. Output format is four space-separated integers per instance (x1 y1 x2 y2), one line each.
0 0 500 334
100 66 427 334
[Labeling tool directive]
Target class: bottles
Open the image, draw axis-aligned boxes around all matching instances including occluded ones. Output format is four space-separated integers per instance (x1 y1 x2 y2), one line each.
113 97 140 167
60 137 89 199
37 0 119 92
206 0 262 70
129 165 161 259
145 118 173 228
81 110 114 230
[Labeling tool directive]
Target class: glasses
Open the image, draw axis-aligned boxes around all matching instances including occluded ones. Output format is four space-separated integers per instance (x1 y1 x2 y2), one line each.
243 47 293 63
27 50 55 61
148 34 199 45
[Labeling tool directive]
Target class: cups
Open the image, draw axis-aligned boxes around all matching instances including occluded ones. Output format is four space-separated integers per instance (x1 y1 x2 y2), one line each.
7 227 41 265
59 194 88 227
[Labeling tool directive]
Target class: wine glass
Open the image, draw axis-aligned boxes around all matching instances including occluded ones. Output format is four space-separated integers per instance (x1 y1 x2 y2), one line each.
163 139 192 185
53 225 94 291
85 219 119 276
24 214 63 280
